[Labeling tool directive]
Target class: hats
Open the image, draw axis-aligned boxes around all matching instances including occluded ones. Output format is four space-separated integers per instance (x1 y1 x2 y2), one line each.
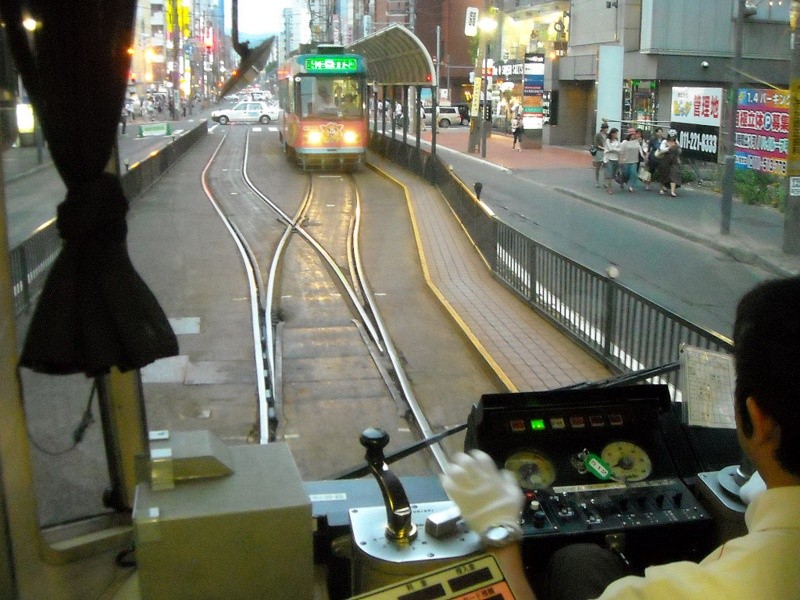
668 130 677 137
628 128 636 134
600 123 608 128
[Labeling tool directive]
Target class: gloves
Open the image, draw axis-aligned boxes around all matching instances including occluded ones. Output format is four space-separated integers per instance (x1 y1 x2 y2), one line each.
440 449 522 535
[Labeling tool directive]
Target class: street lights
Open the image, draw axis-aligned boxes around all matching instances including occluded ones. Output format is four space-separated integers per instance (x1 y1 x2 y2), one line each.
480 16 497 157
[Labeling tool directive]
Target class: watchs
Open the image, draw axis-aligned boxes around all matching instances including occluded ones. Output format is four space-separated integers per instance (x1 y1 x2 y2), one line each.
476 520 523 550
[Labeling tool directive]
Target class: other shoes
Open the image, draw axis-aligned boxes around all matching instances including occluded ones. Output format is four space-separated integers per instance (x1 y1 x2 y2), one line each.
672 192 677 197
607 189 613 194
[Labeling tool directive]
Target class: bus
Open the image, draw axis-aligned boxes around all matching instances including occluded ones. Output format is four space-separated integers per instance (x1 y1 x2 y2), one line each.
277 44 367 173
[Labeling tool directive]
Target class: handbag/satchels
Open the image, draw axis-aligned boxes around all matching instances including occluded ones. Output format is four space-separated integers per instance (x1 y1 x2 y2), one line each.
615 169 624 183
589 145 597 155
638 167 651 184
654 149 665 159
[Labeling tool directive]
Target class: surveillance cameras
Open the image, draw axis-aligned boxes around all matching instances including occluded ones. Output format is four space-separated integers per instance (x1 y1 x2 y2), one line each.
700 60 710 69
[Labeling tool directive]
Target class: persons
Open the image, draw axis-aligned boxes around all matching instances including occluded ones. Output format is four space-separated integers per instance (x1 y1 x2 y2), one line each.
118 91 212 136
378 99 440 134
319 94 336 113
593 121 665 194
438 272 800 600
510 113 524 149
652 129 684 196
339 94 358 113
333 86 344 103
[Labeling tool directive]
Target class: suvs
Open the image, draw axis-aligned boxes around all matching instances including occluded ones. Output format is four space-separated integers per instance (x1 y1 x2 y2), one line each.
423 104 471 128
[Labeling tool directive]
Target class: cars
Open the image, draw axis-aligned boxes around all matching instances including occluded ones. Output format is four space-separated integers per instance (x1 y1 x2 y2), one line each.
224 87 271 101
211 100 278 124
